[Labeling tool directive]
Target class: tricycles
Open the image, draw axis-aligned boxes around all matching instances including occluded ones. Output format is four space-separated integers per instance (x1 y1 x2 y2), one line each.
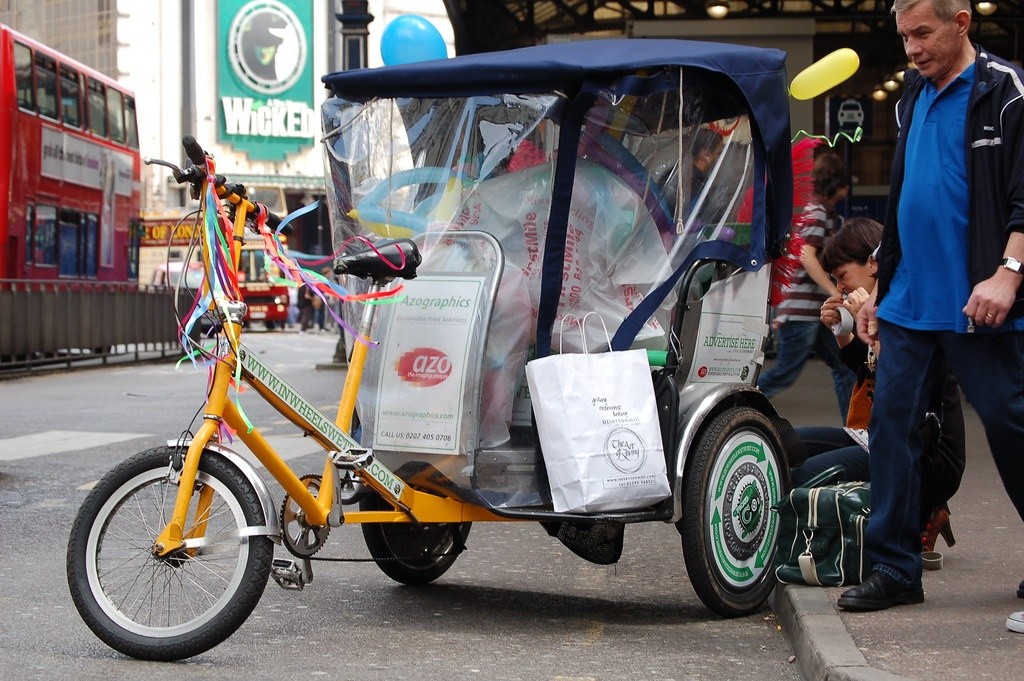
66 32 793 663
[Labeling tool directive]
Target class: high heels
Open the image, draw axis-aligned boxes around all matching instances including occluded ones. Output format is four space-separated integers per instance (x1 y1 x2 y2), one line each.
922 508 955 553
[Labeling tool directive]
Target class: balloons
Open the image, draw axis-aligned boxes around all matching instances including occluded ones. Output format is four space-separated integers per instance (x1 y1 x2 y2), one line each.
346 94 673 366
790 47 859 100
380 14 448 67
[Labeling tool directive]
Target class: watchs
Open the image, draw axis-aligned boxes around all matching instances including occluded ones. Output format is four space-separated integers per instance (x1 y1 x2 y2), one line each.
999 257 1024 276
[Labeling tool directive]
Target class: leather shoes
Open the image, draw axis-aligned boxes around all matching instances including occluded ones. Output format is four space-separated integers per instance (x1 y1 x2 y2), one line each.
1017 580 1024 597
837 570 924 610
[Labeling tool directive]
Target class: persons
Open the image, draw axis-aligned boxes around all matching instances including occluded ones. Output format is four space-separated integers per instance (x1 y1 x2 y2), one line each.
756 153 854 425
838 0 1024 610
287 267 340 335
657 130 736 241
793 218 884 490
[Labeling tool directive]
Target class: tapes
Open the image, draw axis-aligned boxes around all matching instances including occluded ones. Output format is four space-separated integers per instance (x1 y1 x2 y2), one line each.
830 306 854 337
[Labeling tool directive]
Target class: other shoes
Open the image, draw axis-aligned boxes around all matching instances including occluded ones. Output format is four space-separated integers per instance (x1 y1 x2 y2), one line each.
1006 611 1024 634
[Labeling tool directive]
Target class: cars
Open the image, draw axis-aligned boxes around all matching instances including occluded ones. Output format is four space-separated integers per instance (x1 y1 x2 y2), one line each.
153 261 225 337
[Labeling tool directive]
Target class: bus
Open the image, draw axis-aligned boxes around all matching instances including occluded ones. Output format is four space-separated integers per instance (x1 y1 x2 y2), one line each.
228 242 295 332
1 23 148 370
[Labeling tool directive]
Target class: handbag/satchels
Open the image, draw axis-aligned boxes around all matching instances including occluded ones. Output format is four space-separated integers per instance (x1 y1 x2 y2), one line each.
771 480 872 586
525 311 672 513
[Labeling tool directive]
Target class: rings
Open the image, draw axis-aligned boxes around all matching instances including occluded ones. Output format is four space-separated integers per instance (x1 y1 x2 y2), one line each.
986 314 994 318
